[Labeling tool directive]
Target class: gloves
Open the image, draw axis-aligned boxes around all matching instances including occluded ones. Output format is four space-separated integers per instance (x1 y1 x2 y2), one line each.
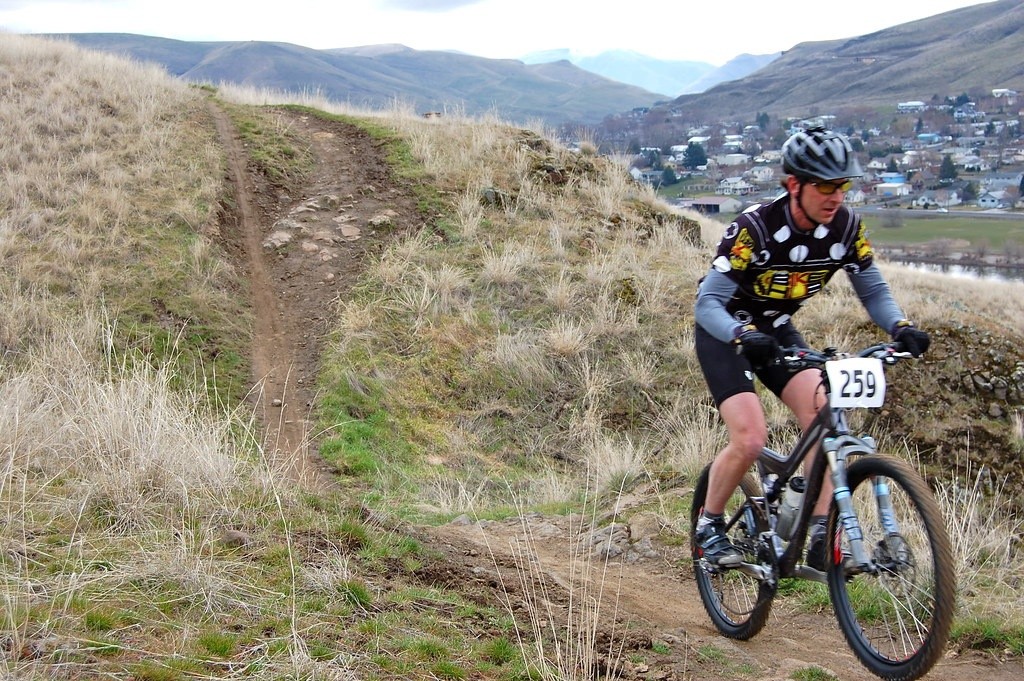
729 324 781 367
890 320 931 359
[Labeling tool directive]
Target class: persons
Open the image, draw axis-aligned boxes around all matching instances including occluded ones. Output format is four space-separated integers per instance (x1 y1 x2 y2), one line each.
694 127 931 575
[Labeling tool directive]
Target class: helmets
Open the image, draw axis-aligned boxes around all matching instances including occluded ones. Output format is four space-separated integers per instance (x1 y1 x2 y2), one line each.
780 126 865 181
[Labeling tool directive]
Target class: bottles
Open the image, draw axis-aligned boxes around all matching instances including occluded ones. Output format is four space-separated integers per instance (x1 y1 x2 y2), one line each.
775 475 807 542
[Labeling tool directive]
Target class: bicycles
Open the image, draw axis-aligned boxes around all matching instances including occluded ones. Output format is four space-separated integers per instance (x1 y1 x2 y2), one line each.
688 335 959 681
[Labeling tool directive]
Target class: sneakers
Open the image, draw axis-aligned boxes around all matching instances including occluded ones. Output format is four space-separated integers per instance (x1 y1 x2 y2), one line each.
806 530 851 573
692 520 744 565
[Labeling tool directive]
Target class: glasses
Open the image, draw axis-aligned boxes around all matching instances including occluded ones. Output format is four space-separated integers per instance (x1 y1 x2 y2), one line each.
797 180 853 195
